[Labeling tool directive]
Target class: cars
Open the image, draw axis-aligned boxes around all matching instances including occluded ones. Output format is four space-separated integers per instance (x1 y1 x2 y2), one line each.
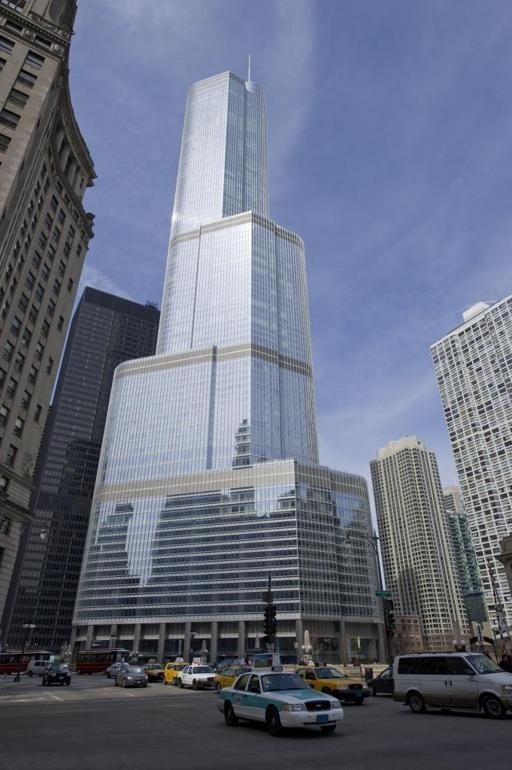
104 663 130 677
115 667 147 688
215 658 242 669
218 671 343 737
368 666 393 696
180 665 219 691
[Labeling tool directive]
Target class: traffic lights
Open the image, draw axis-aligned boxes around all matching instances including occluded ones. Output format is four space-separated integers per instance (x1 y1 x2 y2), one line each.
388 614 396 631
263 606 270 634
270 605 278 634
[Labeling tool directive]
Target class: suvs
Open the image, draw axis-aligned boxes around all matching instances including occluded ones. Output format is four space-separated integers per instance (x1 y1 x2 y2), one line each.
213 667 258 690
297 667 374 706
42 661 73 686
145 663 164 681
164 663 188 687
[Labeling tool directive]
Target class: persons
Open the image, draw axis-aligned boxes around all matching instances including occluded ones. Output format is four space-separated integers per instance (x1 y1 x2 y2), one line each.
501 650 512 673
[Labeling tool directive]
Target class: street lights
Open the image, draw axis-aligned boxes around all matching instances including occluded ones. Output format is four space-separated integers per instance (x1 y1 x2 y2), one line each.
15 623 36 682
0 509 48 540
454 640 467 651
344 527 395 667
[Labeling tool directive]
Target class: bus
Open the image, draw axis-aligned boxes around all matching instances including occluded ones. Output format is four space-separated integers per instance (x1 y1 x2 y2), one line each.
0 651 52 676
251 652 298 672
75 647 130 674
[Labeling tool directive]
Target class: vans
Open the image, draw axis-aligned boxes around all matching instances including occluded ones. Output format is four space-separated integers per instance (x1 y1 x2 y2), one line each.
392 650 512 718
26 660 52 676
